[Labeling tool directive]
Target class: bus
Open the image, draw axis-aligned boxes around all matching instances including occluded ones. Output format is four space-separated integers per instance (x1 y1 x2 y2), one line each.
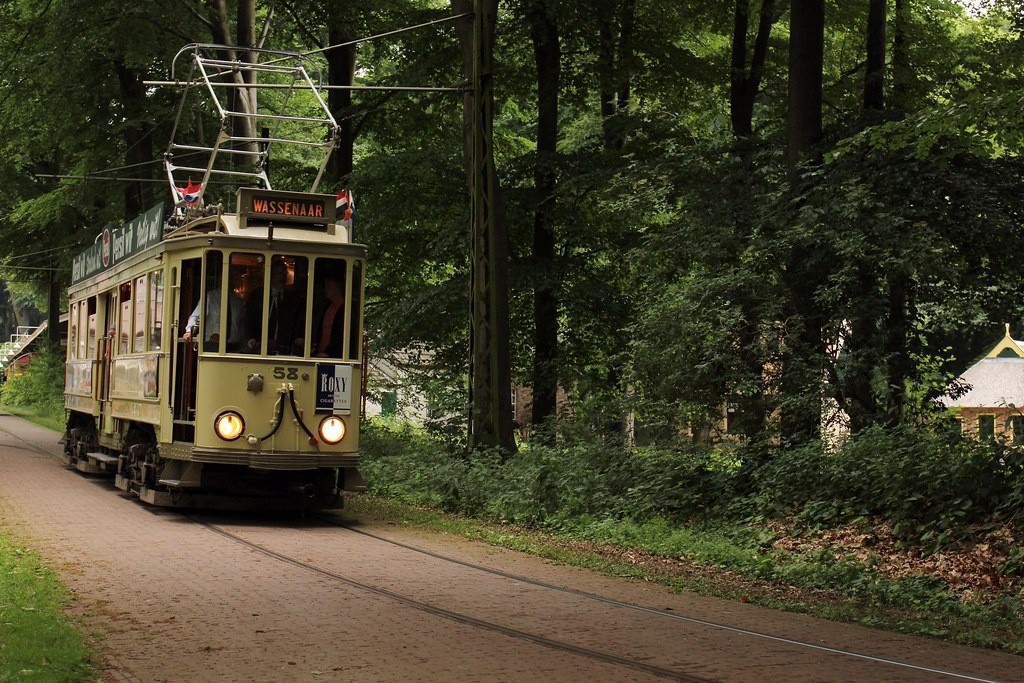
58 41 368 510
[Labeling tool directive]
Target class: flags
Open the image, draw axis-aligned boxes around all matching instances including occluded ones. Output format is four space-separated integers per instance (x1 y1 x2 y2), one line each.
173 180 204 206
336 189 356 221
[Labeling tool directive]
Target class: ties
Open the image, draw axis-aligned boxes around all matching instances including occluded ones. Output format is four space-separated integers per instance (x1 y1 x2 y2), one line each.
227 300 232 341
276 290 284 318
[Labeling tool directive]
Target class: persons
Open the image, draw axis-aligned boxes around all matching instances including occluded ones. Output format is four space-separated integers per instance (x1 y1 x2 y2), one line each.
269 257 295 341
268 272 356 358
183 266 263 354
